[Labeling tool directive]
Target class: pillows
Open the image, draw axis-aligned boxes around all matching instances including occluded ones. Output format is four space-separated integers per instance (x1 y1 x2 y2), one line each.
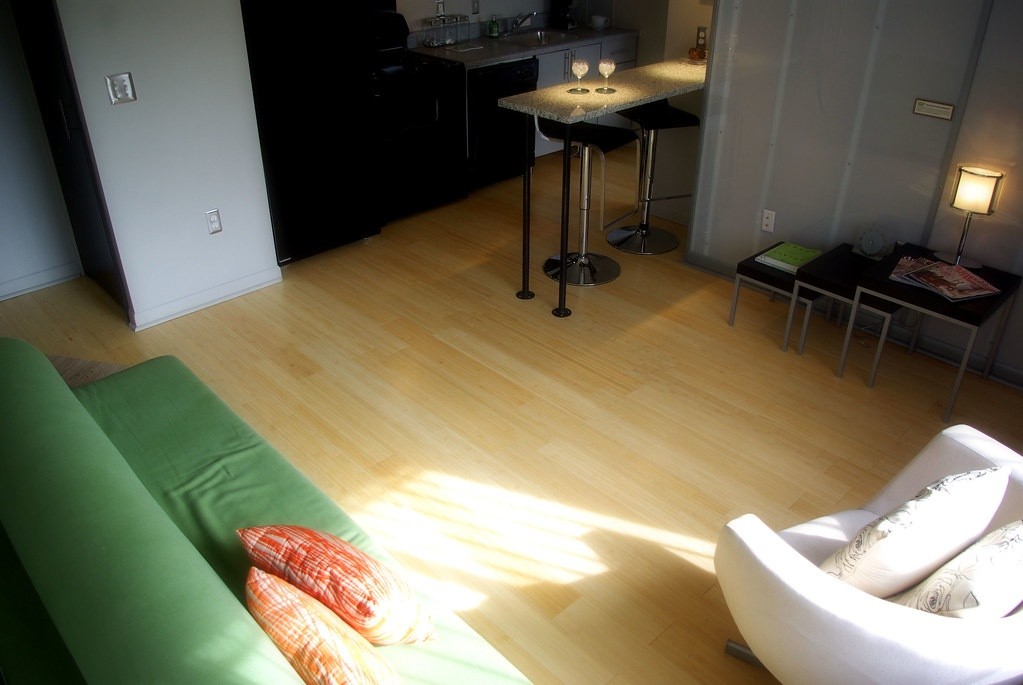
244 567 418 685
888 520 1023 620
237 526 442 648
819 462 1012 597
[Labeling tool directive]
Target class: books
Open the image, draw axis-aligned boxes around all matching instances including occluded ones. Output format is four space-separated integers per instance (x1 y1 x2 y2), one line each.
889 256 1001 303
754 241 822 276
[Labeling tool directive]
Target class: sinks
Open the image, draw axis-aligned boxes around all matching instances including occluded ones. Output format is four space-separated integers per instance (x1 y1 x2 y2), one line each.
502 31 576 47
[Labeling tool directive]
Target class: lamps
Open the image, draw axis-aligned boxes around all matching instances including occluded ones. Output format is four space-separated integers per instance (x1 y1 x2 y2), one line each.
950 162 1007 265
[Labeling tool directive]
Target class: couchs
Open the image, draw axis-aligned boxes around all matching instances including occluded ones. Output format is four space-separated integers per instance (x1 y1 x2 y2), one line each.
0 333 533 685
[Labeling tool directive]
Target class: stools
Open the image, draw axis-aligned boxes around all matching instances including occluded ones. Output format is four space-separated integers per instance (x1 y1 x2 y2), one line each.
533 113 640 288
605 97 701 256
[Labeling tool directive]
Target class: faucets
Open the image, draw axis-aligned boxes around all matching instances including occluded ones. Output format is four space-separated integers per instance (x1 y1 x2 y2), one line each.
511 10 536 31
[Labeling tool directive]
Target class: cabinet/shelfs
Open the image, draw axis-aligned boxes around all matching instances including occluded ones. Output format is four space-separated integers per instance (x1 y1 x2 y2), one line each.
422 36 639 194
239 1 424 268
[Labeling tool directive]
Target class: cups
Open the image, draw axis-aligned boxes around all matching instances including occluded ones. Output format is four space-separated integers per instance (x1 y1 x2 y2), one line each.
591 15 609 28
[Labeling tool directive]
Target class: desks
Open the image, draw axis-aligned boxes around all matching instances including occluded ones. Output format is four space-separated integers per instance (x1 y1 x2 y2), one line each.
835 243 1023 422
781 242 924 387
498 58 707 317
729 241 846 354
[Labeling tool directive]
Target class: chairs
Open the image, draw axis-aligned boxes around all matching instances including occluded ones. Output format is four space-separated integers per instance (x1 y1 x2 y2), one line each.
714 423 1023 685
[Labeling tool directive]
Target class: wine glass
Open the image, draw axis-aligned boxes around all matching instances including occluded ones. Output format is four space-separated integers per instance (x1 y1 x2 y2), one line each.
567 58 590 94
595 59 618 94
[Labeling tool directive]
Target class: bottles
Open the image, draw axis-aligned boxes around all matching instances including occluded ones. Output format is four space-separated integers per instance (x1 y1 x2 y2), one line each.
488 15 499 37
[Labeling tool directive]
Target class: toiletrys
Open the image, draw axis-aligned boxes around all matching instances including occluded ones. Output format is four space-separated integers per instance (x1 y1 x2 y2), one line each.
489 16 499 37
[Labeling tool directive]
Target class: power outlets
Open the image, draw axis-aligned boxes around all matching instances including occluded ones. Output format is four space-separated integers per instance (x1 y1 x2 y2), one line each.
207 208 223 235
